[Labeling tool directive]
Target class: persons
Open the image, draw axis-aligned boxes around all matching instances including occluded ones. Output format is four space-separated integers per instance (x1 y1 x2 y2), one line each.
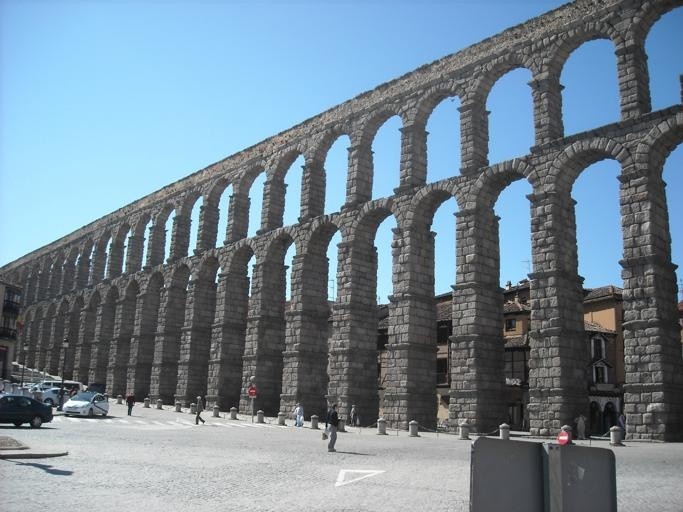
68 385 77 399
604 411 627 431
573 411 588 439
56 383 65 404
83 387 89 392
348 404 359 427
126 391 135 416
291 403 298 426
325 404 342 452
295 402 304 427
31 385 42 402
195 395 205 425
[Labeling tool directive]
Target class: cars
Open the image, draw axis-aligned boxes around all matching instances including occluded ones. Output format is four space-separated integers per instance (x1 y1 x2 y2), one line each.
0 393 53 429
32 388 71 408
62 392 109 417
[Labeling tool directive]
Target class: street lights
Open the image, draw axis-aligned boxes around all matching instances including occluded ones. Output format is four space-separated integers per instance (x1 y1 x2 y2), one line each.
57 337 69 410
20 342 29 387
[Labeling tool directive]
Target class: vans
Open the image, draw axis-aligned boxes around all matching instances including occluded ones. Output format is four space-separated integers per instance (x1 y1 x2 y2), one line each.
29 380 84 394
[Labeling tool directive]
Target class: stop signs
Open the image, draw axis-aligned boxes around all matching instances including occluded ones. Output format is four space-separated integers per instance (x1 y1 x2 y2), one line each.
248 386 257 397
558 430 569 445
